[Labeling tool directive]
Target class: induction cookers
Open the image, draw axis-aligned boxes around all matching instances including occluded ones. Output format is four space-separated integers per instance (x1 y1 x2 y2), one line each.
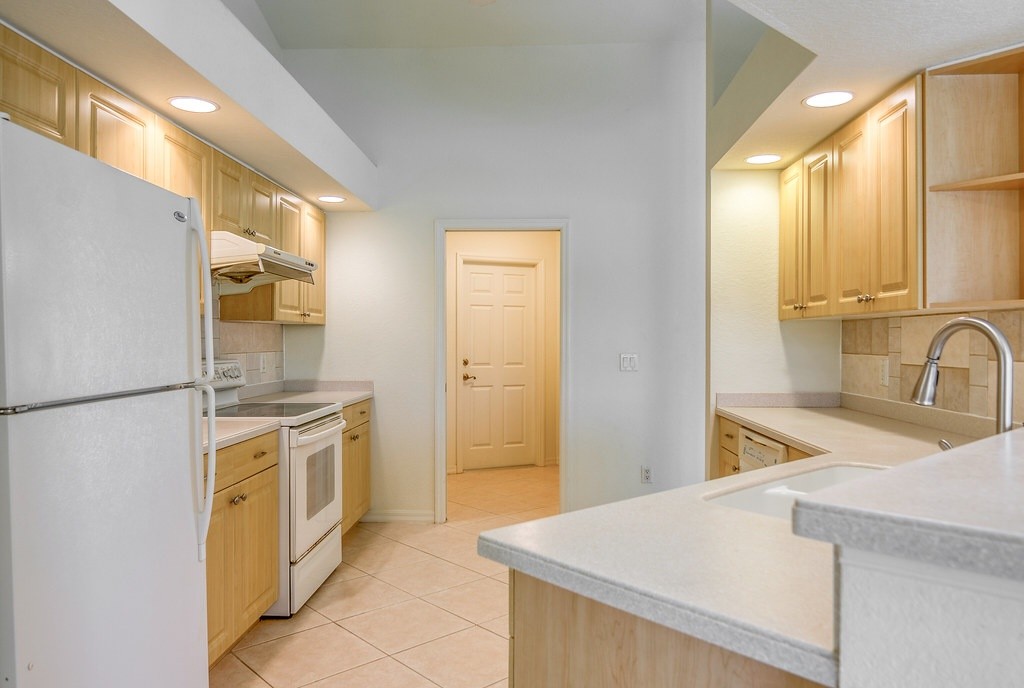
203 360 343 426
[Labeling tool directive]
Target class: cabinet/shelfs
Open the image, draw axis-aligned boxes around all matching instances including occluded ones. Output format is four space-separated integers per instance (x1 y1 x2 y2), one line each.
77 69 212 318
833 75 924 321
218 186 325 325
719 416 743 478
342 398 371 537
925 41 1024 316
203 430 280 672
212 148 277 248
788 446 814 463
778 136 833 325
509 567 828 688
0 23 76 152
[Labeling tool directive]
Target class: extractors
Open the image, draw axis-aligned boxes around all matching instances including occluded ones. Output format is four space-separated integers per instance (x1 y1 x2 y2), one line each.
210 231 318 295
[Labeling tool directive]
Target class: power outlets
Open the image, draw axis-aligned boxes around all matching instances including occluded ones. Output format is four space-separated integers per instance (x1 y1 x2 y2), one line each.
641 466 654 483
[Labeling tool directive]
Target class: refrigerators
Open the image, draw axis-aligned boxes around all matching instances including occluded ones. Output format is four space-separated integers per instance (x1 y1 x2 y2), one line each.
0 112 216 688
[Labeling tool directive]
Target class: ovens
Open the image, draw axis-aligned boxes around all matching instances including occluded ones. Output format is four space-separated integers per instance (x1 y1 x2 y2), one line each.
261 413 347 620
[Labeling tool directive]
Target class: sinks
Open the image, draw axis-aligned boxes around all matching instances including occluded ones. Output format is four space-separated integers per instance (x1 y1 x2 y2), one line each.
702 463 893 531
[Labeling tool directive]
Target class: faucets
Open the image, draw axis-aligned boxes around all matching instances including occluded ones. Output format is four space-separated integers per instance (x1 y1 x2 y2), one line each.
909 316 1014 435
